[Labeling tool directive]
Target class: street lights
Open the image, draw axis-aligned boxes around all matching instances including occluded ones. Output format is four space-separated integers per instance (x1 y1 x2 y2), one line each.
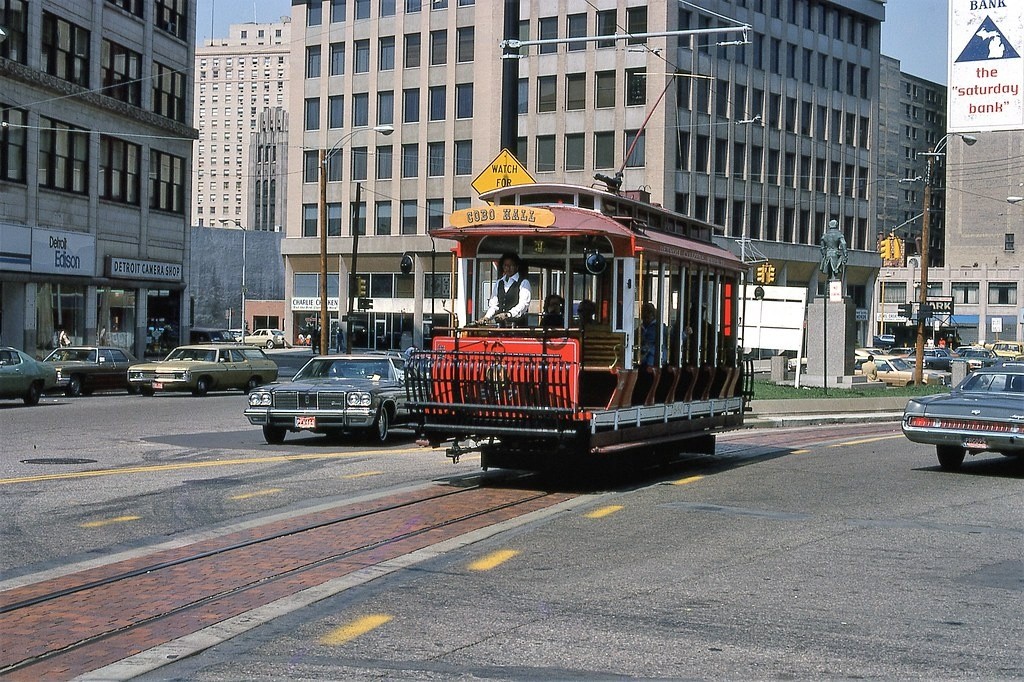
216 219 246 342
317 126 395 376
914 135 978 388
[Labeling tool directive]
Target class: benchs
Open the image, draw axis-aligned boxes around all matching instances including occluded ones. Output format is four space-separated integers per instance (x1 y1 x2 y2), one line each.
464 327 625 372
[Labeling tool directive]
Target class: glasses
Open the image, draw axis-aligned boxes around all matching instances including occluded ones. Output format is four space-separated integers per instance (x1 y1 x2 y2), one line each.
577 309 591 312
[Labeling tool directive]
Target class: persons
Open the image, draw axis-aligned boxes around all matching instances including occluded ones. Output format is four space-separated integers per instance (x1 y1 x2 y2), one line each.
310 329 321 354
927 336 934 347
298 333 304 345
100 325 105 346
55 325 70 355
820 219 848 295
336 329 344 353
478 252 532 328
939 337 946 348
539 295 565 327
307 334 311 346
669 303 712 364
862 356 877 377
577 300 597 324
634 302 667 367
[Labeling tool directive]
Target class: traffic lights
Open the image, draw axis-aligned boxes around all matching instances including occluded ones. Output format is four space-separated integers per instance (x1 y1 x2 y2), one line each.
757 264 777 284
898 304 912 318
920 305 934 319
358 299 372 309
356 277 367 297
880 239 892 260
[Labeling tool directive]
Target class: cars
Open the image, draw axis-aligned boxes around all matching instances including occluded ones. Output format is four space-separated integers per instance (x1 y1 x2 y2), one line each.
127 345 279 397
43 347 152 397
189 328 285 349
855 334 1024 387
901 367 1024 467
243 351 432 444
0 347 56 406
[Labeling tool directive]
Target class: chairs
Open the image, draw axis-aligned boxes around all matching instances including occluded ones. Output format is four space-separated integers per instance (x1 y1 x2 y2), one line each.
1004 377 1024 393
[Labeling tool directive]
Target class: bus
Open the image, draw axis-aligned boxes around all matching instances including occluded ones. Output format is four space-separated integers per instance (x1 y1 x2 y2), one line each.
404 76 756 477
